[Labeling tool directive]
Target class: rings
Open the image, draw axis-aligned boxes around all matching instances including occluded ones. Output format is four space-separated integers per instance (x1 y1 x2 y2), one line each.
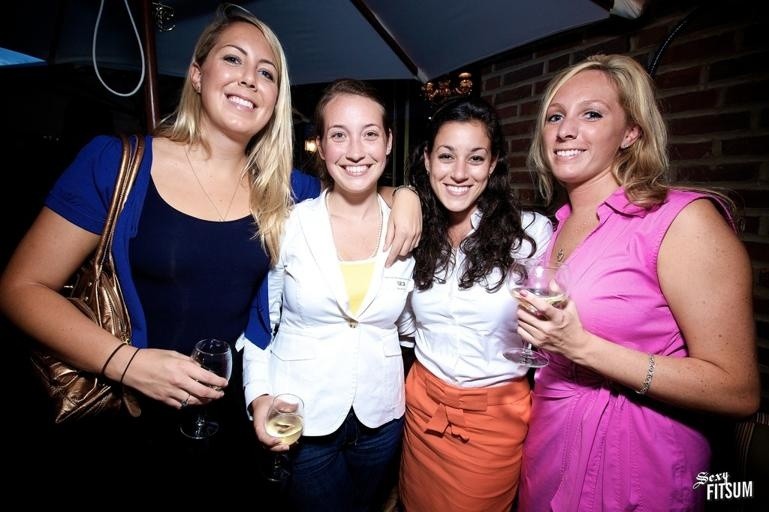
178 393 193 407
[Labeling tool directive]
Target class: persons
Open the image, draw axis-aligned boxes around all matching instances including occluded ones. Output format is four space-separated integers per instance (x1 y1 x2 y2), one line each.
390 97 559 511
233 76 426 512
0 2 426 511
508 47 763 512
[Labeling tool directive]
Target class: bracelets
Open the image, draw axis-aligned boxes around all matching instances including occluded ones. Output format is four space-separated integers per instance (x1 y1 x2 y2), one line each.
635 351 658 402
115 346 147 385
386 181 422 206
97 340 130 377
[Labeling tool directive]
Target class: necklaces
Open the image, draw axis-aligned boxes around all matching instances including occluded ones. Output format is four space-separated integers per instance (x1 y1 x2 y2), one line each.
552 226 572 266
180 138 248 228
321 188 386 263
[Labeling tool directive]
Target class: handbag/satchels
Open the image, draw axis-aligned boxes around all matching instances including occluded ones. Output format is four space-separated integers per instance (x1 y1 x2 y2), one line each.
28 131 145 425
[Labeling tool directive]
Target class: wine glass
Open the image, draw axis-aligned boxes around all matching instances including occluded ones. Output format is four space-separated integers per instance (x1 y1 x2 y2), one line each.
261 392 308 487
501 256 574 369
179 336 233 441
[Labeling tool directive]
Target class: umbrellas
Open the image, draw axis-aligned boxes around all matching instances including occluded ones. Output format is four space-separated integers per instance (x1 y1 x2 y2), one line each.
0 0 619 137
